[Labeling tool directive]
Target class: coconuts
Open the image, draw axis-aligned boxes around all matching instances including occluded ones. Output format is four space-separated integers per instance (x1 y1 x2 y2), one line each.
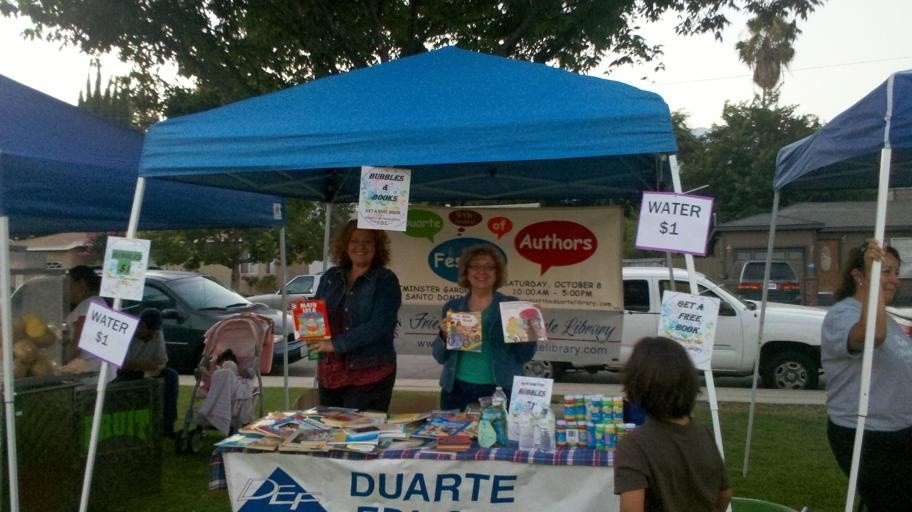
12 314 55 380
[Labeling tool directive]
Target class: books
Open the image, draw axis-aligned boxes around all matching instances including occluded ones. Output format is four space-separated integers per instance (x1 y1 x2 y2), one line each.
444 310 483 354
290 300 332 343
210 398 492 453
499 300 548 345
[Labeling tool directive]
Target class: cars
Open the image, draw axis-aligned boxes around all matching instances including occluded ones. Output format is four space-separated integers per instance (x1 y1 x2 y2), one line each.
90 268 312 374
885 306 912 339
520 264 835 389
248 274 323 311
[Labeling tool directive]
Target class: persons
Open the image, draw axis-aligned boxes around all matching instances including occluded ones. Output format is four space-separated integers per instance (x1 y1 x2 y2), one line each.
198 348 239 391
432 243 539 414
298 218 403 412
819 240 912 512
65 264 110 364
613 335 733 512
118 308 184 438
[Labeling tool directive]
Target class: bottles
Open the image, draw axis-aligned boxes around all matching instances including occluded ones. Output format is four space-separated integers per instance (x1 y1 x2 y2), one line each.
554 393 637 451
537 402 557 454
518 402 537 450
492 386 506 410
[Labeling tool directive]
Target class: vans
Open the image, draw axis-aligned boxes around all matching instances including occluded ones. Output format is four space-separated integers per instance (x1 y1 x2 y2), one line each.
730 260 802 303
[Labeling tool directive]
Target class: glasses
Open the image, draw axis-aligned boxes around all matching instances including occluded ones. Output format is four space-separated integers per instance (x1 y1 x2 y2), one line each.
469 263 495 271
